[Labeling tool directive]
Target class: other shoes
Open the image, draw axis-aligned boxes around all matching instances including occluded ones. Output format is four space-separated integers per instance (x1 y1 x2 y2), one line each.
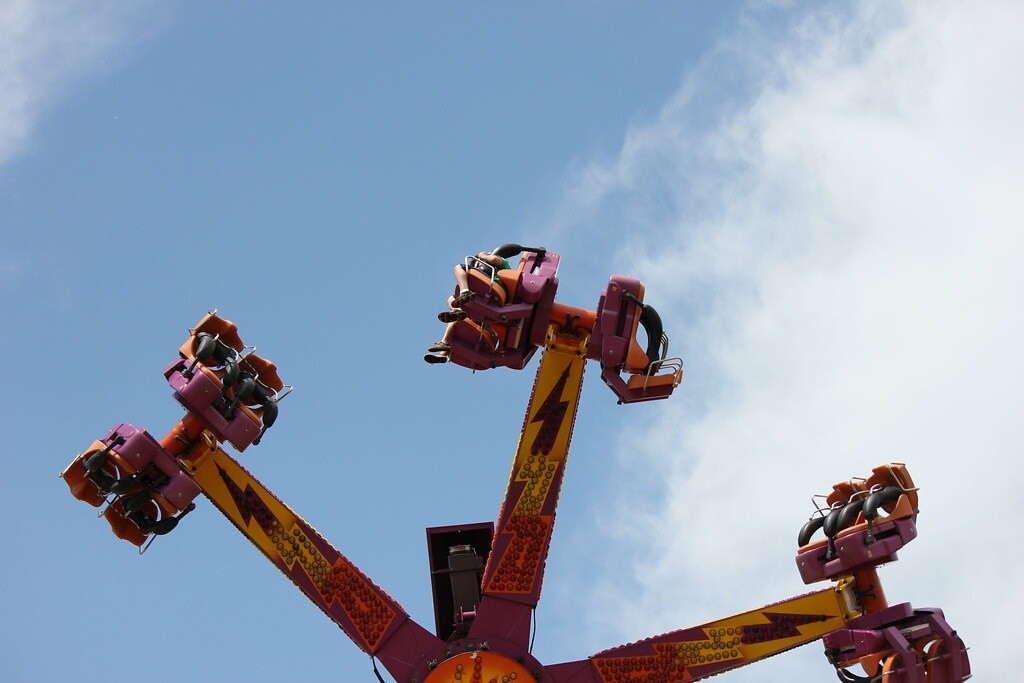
451 290 476 308
428 342 451 352
438 310 467 323
424 354 447 363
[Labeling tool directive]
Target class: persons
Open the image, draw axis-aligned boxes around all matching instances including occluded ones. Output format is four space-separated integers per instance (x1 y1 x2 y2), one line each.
424 320 457 364
437 250 512 323
